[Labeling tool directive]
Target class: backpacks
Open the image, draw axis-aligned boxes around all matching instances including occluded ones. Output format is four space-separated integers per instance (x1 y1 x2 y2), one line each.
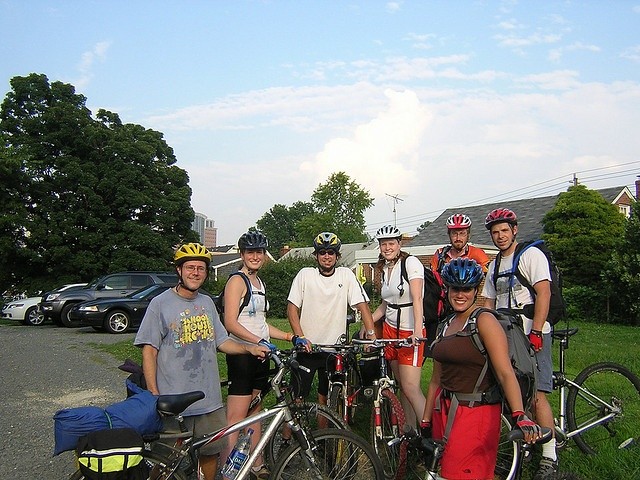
379 254 450 358
422 307 541 443
493 239 568 345
211 271 269 353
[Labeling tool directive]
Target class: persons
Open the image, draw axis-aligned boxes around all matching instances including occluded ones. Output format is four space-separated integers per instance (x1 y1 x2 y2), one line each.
359 224 427 436
277 232 377 470
133 243 271 480
223 230 312 480
428 213 491 277
481 208 559 480
420 256 542 480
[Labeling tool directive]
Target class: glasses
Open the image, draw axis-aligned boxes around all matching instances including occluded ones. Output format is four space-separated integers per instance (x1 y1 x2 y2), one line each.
318 249 336 255
182 265 206 273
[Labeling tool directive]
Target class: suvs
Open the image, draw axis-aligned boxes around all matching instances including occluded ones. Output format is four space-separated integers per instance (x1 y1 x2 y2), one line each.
40 270 179 328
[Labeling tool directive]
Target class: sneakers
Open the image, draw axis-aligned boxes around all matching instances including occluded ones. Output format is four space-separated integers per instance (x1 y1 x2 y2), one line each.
277 435 292 459
249 463 275 480
532 456 559 480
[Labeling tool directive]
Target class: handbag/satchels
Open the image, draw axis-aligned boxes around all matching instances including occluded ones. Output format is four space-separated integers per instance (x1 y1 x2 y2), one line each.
75 428 149 480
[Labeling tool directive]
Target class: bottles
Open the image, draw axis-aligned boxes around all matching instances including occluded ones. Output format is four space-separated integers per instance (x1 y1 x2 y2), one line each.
219 428 254 479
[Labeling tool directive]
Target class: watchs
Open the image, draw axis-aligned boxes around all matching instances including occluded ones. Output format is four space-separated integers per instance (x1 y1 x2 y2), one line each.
365 329 376 336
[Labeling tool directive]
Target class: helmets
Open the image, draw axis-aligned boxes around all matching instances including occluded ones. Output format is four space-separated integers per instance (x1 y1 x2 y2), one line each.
439 257 485 289
485 207 518 230
312 232 341 253
174 242 214 268
375 224 402 239
446 213 472 229
238 230 270 249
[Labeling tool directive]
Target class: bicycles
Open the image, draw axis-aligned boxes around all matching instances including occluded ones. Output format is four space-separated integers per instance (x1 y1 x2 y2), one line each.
387 424 553 480
117 343 357 480
66 351 385 480
313 314 363 479
349 336 429 480
495 326 640 480
293 333 369 480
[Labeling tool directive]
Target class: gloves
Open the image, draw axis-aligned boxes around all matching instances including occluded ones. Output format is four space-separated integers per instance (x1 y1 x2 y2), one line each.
528 329 543 350
292 335 307 352
511 410 542 436
419 420 433 439
256 338 277 354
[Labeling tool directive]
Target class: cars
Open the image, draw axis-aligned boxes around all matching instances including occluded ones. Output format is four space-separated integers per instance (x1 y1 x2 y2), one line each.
1 283 114 326
69 283 218 334
